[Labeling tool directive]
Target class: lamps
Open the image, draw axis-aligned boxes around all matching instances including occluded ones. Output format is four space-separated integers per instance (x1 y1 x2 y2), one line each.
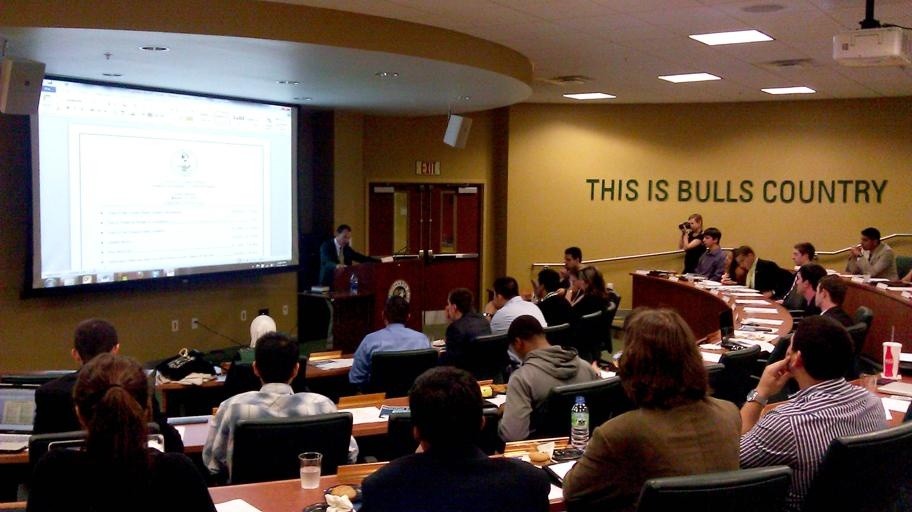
581 310 602 361
474 333 513 376
228 413 353 484
632 466 793 512
600 301 616 364
751 334 791 404
383 408 499 459
371 348 439 398
854 306 874 331
896 254 912 280
719 344 761 392
707 364 725 398
799 420 912 512
847 322 867 354
222 357 306 404
534 376 624 437
607 291 621 323
779 267 796 298
542 323 570 350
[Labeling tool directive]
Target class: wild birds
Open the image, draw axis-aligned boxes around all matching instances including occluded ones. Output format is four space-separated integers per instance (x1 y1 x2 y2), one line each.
48 434 165 456
0 383 41 453
719 308 746 348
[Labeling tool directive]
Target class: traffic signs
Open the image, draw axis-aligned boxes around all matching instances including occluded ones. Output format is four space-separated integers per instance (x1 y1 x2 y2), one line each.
282 305 288 316
192 318 199 330
171 320 180 332
240 310 247 321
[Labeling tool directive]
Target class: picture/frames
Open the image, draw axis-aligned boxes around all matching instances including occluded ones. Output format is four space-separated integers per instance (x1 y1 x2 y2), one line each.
333 259 422 352
0 272 912 511
156 340 445 411
1 273 793 463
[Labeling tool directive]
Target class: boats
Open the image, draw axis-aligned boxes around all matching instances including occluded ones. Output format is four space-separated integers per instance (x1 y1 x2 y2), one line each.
0 57 46 114
443 114 473 150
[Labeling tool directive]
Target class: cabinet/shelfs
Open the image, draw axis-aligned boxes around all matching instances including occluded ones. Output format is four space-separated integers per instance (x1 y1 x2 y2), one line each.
571 397 590 454
348 272 358 297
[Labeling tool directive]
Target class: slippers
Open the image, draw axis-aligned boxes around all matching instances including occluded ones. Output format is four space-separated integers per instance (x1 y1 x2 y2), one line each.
165 345 217 384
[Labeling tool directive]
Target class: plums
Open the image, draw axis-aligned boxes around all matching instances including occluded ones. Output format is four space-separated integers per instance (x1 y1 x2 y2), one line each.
833 26 912 67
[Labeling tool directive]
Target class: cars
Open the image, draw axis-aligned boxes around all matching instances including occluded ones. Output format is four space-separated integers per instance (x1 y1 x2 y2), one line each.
860 375 876 392
863 272 870 283
298 452 323 489
883 342 902 378
687 273 694 283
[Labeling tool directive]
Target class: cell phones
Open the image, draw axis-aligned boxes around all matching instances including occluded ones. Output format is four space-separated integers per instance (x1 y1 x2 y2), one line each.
679 222 690 229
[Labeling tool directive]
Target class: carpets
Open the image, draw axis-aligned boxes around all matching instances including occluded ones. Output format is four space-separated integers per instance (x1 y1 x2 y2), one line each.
339 245 344 265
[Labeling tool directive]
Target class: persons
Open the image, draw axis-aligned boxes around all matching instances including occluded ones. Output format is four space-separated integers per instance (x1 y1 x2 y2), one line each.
476 274 548 338
349 368 553 511
734 245 790 301
565 270 584 296
560 310 745 512
203 332 361 472
441 288 494 371
733 313 888 504
675 213 703 274
847 227 901 280
229 316 275 387
346 295 434 392
722 250 739 282
695 228 728 283
566 266 612 314
494 314 608 445
790 274 857 340
36 318 120 437
772 242 821 306
318 224 385 295
560 246 585 286
33 353 216 511
528 269 579 326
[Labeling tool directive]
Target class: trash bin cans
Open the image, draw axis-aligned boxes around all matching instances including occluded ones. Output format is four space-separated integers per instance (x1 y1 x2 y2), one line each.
742 389 767 413
768 286 781 300
855 249 866 266
481 306 494 323
